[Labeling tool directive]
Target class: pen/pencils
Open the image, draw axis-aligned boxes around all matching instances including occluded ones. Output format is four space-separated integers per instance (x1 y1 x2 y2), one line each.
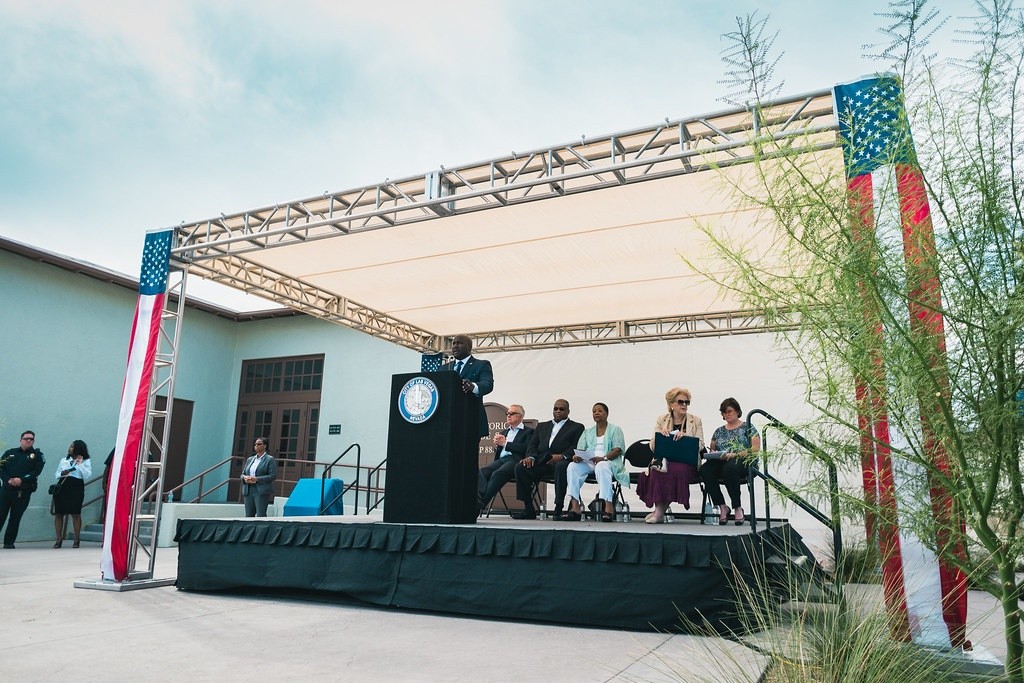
707 446 712 450
465 381 472 387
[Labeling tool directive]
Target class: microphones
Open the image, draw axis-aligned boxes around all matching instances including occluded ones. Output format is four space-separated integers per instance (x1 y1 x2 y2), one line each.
449 356 454 371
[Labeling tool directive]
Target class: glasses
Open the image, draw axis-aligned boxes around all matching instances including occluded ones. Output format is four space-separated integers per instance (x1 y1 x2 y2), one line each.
253 443 263 446
21 437 34 441
554 407 565 411
505 412 520 415
671 400 690 405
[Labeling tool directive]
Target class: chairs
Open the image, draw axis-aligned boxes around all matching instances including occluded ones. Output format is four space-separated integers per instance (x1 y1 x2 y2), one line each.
480 440 758 527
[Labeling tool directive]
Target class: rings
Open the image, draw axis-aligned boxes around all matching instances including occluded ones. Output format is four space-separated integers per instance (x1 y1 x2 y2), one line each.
466 383 468 385
468 385 470 386
681 435 682 436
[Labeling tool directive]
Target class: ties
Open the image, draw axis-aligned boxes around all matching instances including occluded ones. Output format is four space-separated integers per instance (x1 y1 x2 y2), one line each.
456 361 463 374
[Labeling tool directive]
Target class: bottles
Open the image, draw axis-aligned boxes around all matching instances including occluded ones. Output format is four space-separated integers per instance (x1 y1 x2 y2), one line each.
540 500 546 521
579 501 586 521
622 502 631 522
167 491 173 502
615 501 623 522
704 502 714 525
712 505 721 525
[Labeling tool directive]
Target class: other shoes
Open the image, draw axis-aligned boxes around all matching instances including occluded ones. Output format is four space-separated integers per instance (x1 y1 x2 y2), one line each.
734 508 745 525
719 506 731 525
552 514 562 520
644 512 664 524
512 510 536 519
3 544 15 548
73 542 79 547
601 512 612 522
561 511 581 520
53 538 62 548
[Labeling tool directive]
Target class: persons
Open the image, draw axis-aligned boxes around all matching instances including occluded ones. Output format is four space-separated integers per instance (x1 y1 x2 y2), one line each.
477 405 534 518
240 437 276 517
636 389 704 523
561 403 630 522
513 399 585 520
53 440 91 547
0 431 46 549
438 335 494 439
699 398 760 526
102 447 115 490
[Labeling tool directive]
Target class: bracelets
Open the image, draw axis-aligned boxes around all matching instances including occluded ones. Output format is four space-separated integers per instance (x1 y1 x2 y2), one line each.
604 456 607 461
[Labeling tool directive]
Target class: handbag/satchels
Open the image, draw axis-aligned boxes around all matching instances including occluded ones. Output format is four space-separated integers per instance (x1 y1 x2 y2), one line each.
703 451 724 459
48 484 62 495
